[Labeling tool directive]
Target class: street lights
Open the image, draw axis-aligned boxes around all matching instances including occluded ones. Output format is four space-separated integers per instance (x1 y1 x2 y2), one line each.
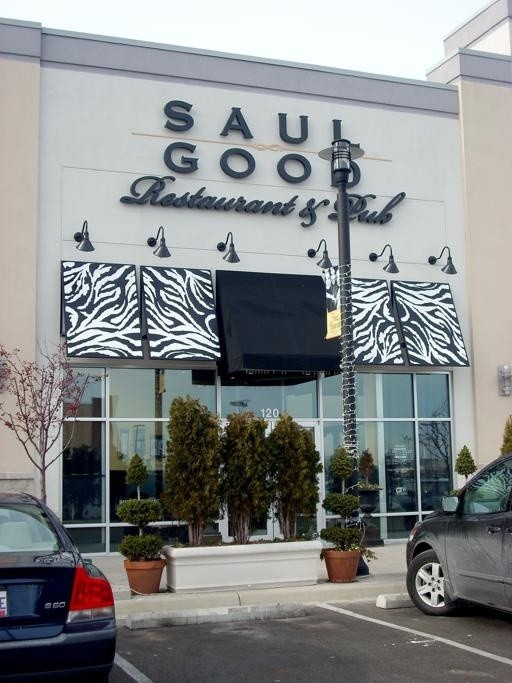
328 134 378 585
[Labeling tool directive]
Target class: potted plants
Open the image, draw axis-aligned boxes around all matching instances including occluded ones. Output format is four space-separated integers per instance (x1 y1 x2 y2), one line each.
318 444 380 584
112 451 169 599
152 388 331 595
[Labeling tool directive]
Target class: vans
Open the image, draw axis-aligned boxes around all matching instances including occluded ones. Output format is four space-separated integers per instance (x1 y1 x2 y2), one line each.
393 487 407 497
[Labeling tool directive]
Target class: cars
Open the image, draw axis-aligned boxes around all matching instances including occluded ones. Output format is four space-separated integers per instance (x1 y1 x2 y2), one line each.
402 448 512 620
403 476 450 529
0 490 122 683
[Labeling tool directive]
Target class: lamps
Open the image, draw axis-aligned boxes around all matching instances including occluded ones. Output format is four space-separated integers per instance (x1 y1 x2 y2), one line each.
307 237 333 270
73 217 96 253
217 230 240 263
146 224 172 258
427 244 458 274
367 242 399 273
494 361 511 397
